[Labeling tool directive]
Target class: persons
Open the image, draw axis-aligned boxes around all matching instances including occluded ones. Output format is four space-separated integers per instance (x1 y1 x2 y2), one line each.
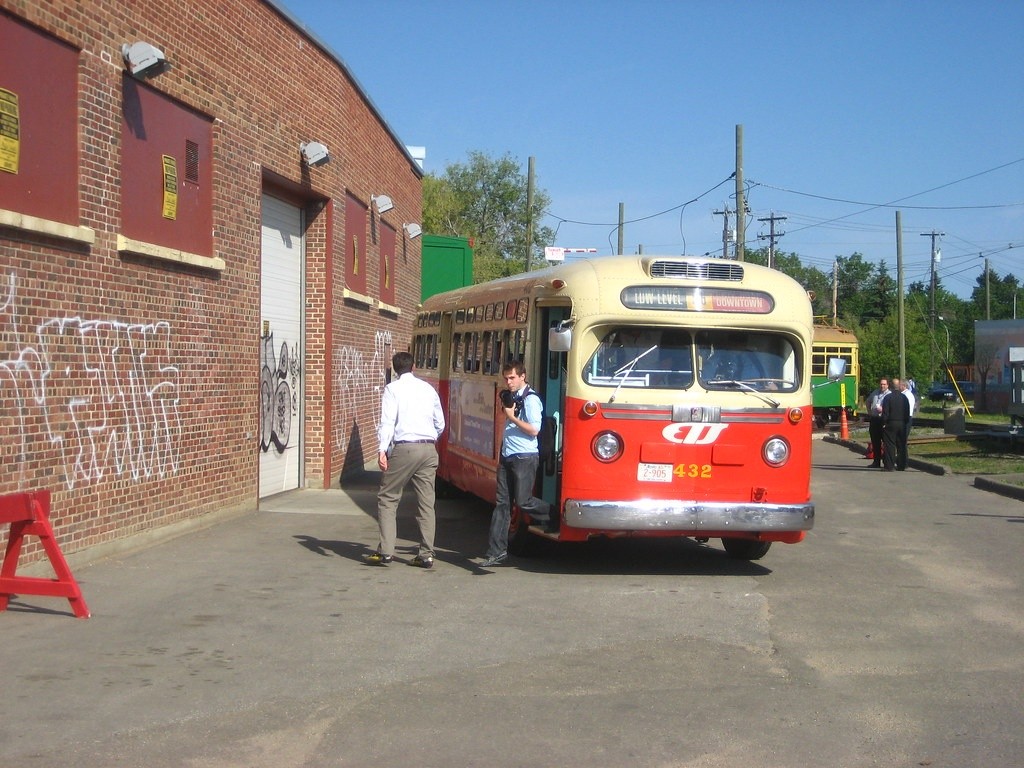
880 377 917 472
478 361 560 567
364 352 445 570
865 377 892 469
700 332 779 391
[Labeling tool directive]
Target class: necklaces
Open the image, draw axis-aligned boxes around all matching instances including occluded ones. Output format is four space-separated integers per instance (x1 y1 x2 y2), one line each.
902 390 908 394
877 389 889 404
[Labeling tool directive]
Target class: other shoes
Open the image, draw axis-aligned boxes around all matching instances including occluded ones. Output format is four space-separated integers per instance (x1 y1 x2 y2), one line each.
880 466 894 472
544 513 561 533
896 467 906 471
478 552 508 567
868 463 881 468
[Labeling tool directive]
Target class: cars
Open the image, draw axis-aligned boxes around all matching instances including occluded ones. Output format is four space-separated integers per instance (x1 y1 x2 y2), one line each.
929 380 975 404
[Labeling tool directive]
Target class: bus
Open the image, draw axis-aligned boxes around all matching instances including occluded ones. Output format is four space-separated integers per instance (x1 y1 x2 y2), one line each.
404 254 817 560
811 320 862 428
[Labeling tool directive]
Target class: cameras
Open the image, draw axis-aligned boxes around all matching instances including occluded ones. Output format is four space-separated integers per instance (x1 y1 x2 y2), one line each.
499 390 523 417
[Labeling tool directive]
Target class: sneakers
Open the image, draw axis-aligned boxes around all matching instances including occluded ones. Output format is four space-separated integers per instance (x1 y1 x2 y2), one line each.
409 556 434 569
367 552 393 564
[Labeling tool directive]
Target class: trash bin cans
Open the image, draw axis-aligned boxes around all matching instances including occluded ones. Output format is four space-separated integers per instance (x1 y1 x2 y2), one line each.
943 405 965 434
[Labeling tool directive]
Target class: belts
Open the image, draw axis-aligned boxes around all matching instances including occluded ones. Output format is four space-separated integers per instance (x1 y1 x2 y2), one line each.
395 438 435 444
